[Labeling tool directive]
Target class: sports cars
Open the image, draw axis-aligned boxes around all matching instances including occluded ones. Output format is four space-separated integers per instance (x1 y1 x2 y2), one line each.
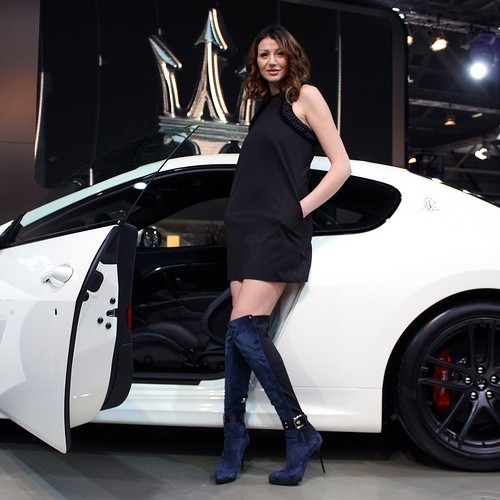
0 153 500 475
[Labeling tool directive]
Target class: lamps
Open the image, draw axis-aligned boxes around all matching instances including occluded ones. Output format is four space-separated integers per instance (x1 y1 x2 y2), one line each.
405 24 499 79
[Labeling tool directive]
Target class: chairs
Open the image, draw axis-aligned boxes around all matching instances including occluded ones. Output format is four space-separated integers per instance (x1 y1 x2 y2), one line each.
127 286 233 370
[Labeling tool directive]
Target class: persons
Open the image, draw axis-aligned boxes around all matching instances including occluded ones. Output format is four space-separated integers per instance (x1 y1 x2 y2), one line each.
213 24 352 486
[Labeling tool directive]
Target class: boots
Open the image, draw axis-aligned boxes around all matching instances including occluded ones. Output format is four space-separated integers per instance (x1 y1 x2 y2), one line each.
214 320 250 485
229 314 325 485
